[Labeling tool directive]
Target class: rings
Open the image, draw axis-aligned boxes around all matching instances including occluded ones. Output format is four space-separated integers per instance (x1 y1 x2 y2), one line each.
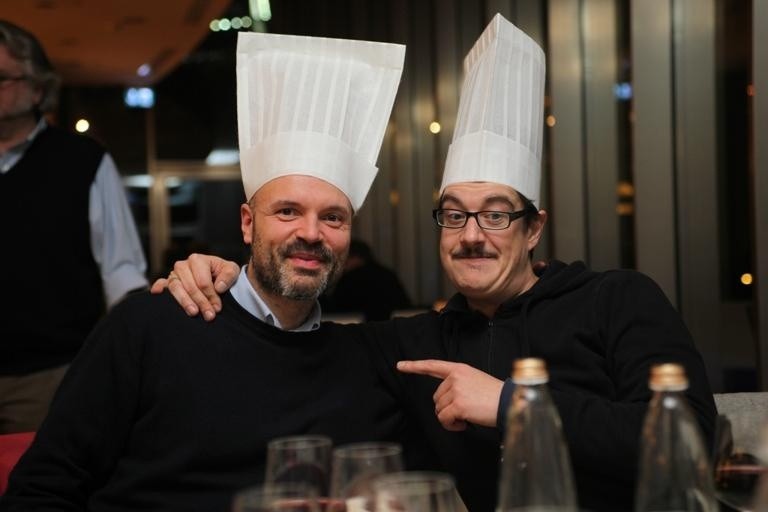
167 276 182 291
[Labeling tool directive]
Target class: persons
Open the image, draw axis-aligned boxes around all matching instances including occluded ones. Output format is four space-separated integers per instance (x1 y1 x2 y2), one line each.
0 173 443 511
1 19 148 436
149 179 742 512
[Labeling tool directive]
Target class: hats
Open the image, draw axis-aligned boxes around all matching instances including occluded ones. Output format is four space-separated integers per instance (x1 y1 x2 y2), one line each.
235 30 408 216
439 12 547 211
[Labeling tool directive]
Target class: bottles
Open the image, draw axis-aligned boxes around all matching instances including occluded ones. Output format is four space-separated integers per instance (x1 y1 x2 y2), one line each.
495 358 580 511
633 362 720 512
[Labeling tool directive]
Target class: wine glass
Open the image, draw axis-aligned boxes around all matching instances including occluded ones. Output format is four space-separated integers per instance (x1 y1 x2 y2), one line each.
231 434 470 512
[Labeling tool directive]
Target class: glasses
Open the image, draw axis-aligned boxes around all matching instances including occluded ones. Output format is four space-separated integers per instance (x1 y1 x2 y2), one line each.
0 74 28 87
432 207 528 230
